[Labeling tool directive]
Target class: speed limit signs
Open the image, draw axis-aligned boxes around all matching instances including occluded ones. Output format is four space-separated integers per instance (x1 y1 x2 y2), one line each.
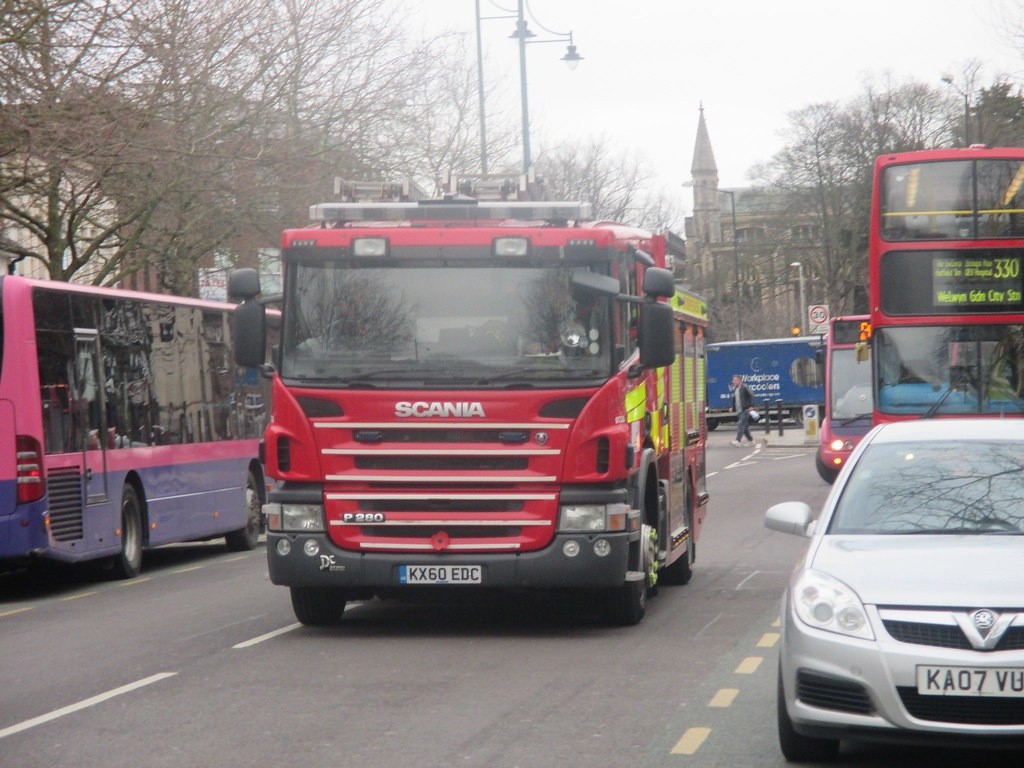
810 306 829 324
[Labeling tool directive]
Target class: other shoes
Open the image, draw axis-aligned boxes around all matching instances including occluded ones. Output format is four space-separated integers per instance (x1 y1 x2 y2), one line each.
744 440 755 447
730 439 742 448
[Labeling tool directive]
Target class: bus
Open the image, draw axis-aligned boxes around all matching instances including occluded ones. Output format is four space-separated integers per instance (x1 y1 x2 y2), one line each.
869 143 1024 424
815 310 878 485
0 275 282 579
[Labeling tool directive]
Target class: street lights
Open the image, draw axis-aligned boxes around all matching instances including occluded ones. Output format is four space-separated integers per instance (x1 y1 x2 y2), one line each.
682 180 741 340
477 11 537 181
789 261 807 336
941 73 968 148
521 31 585 174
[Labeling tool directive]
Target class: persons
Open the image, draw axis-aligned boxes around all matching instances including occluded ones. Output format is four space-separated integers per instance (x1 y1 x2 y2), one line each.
522 270 588 356
986 343 1024 400
729 375 757 448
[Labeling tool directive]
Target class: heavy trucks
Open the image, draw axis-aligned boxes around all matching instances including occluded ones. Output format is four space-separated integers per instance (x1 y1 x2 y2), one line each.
706 335 826 430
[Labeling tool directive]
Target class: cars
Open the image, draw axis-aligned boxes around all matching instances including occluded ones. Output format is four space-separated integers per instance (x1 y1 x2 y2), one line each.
763 416 1024 764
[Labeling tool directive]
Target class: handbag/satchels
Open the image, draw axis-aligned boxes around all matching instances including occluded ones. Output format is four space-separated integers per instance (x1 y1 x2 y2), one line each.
748 410 760 420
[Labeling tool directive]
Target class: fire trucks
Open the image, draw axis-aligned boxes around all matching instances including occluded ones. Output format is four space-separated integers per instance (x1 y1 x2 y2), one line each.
225 176 713 627
